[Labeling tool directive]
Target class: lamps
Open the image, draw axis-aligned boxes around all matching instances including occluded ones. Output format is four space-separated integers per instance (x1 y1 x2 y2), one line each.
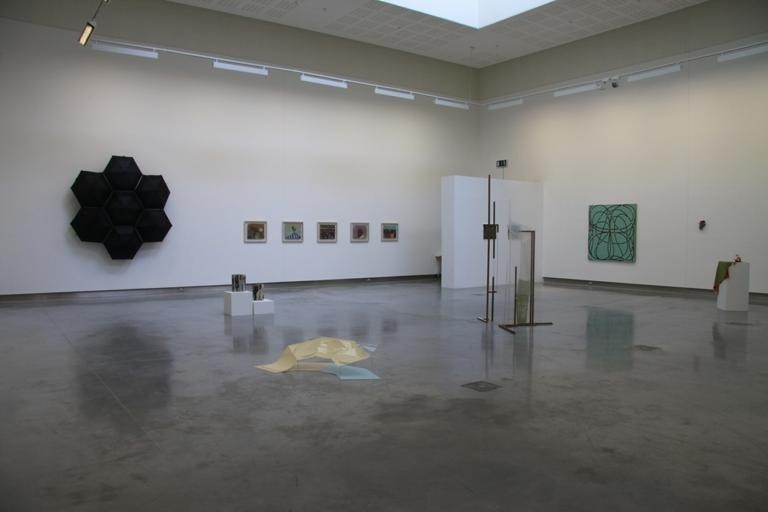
77 0 112 46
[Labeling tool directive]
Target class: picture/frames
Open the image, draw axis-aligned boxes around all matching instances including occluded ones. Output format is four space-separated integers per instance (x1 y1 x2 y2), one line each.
243 220 399 244
